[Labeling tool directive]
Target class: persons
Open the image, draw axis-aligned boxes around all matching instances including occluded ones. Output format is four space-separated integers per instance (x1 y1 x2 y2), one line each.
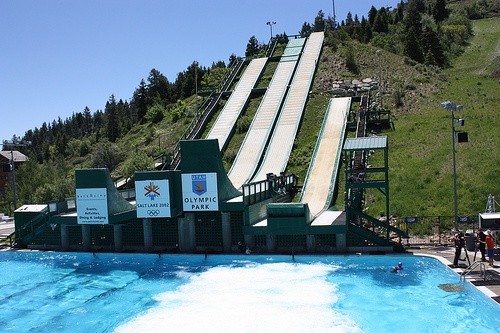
483 229 494 267
394 262 404 270
358 107 364 121
477 228 486 261
350 111 356 122
405 240 410 244
352 163 366 178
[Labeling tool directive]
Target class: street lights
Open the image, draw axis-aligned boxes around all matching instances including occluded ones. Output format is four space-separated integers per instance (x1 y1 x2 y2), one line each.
441 101 468 233
266 21 276 38
376 50 383 108
0 135 32 209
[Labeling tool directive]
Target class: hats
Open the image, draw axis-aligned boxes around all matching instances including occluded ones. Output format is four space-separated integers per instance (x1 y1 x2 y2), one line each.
484 230 490 235
